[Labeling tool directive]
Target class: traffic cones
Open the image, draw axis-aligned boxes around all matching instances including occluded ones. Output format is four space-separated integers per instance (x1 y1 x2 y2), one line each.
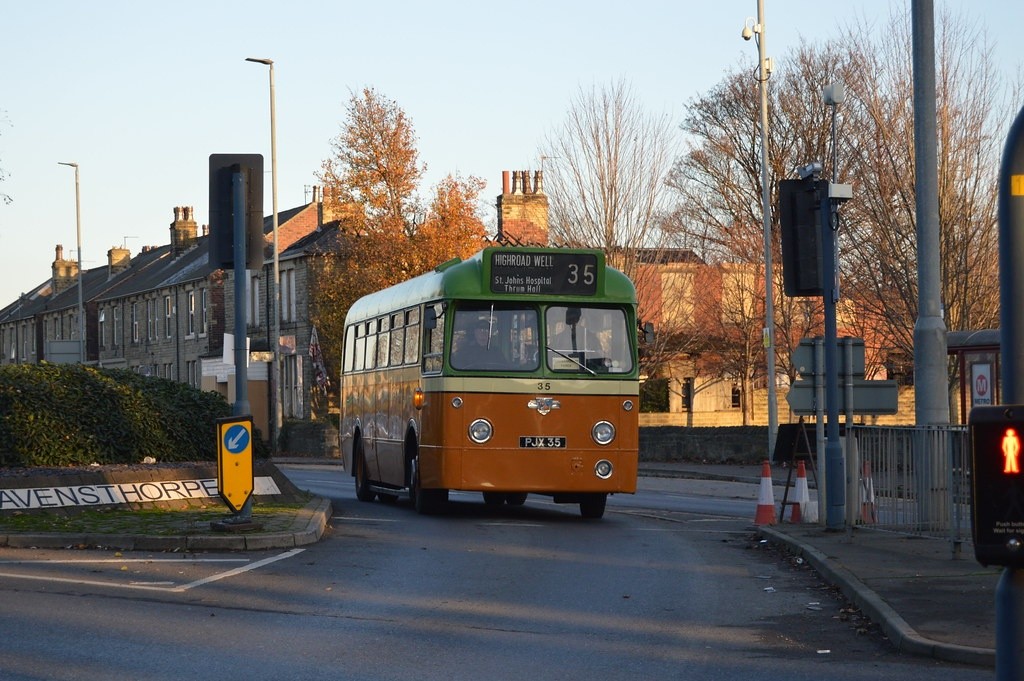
787 460 812 523
860 462 879 526
747 461 778 531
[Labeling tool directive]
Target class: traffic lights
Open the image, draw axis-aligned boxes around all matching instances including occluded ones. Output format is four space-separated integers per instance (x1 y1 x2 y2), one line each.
967 403 1024 570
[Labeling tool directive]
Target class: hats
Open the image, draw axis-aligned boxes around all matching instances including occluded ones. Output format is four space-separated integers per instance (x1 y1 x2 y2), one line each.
476 320 490 329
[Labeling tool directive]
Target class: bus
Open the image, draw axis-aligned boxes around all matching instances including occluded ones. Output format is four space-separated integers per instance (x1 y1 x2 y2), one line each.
339 246 653 519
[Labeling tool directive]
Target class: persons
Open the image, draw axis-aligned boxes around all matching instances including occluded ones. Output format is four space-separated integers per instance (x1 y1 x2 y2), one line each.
456 320 506 367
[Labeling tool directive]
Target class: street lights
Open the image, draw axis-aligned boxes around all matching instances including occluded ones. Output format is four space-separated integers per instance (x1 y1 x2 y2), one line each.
822 84 852 530
58 161 84 364
245 57 277 458
